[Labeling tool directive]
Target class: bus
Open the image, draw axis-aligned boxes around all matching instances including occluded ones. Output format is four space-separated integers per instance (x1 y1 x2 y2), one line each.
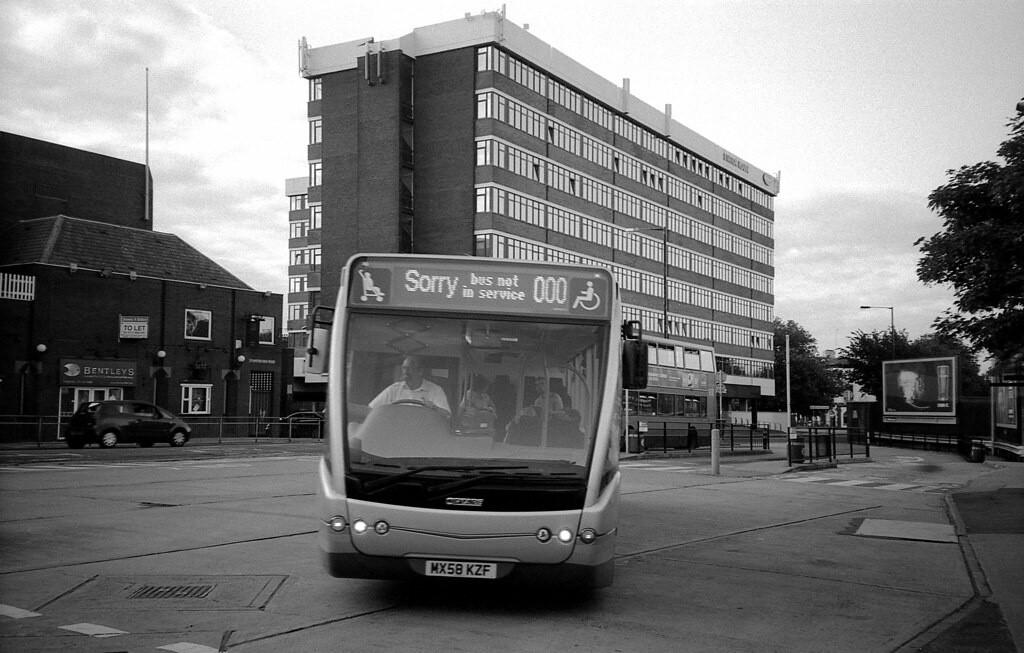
303 252 648 592
615 332 718 452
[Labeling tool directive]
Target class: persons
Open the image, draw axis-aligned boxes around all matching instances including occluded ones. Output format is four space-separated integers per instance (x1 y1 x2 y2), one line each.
461 374 564 420
322 354 452 424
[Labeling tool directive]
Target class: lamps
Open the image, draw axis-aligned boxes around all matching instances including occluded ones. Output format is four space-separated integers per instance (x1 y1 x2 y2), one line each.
153 350 170 378
29 343 46 373
235 355 246 371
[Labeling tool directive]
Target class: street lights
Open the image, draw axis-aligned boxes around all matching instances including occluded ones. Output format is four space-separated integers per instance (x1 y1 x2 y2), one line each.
860 305 895 360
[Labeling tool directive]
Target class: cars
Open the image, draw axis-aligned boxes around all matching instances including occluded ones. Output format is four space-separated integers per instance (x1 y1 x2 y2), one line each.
267 411 325 439
63 400 192 448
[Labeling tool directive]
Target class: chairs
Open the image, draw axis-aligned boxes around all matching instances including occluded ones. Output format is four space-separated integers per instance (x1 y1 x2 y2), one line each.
417 374 584 449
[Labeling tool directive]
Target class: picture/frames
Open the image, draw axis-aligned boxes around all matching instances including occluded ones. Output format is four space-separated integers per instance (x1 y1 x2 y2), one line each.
184 308 211 340
882 356 956 416
